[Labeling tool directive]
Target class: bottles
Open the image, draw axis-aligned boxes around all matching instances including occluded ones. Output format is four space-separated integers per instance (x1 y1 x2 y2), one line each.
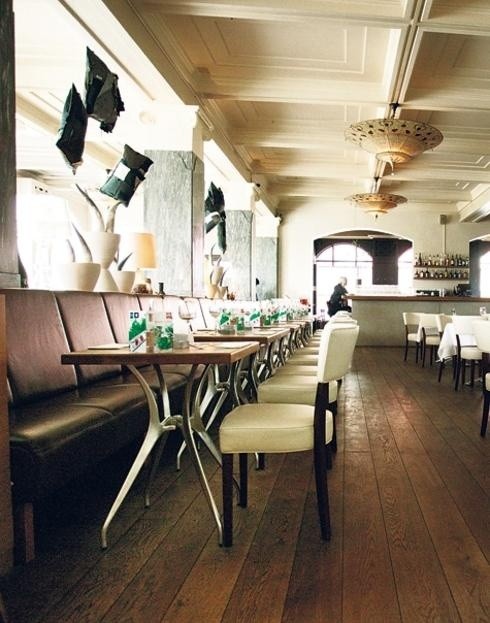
411 251 470 278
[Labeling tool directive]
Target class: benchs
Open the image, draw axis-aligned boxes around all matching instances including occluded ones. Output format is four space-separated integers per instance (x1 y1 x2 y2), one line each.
0 288 292 562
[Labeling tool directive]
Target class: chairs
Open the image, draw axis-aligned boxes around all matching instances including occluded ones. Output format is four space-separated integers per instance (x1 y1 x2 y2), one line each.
219 310 359 547
402 312 490 436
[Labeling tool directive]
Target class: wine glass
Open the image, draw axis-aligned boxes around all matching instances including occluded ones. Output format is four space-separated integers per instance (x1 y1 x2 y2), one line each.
209 306 222 336
479 304 488 318
179 303 197 345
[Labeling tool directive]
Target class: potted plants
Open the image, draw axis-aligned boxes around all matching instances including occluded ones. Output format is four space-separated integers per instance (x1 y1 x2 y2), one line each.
205 244 227 299
67 183 156 294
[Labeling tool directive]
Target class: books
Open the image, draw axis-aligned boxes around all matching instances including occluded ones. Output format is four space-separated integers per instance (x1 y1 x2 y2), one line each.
88 343 129 350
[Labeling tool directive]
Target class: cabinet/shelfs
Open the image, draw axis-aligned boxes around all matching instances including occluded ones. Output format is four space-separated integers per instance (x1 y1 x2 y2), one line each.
413 265 469 280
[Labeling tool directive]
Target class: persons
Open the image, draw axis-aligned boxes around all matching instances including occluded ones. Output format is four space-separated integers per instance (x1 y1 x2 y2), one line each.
326 276 358 316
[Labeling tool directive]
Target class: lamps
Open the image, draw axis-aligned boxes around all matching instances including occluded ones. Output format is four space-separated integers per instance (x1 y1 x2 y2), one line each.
346 103 443 175
344 176 408 218
